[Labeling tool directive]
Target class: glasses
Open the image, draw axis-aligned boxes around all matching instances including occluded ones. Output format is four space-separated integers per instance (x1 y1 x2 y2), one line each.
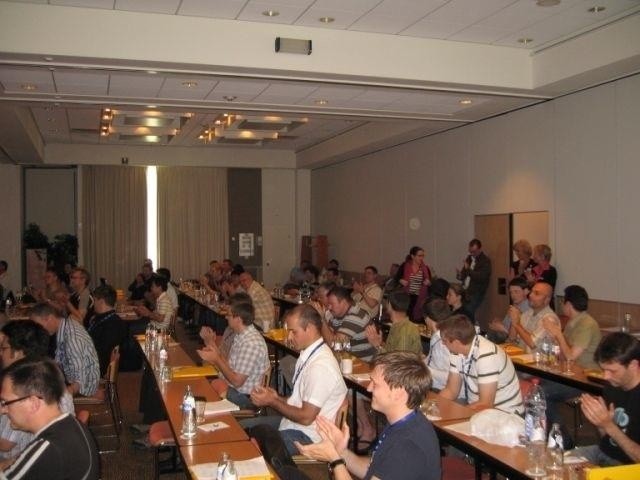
2 393 43 410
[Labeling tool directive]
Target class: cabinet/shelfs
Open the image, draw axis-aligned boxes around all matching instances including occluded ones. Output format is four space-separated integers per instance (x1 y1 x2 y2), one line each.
26 249 47 289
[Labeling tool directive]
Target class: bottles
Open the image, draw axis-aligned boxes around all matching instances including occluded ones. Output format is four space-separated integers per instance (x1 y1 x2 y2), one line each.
282 320 289 341
474 322 487 335
536 334 560 370
331 336 354 375
5 281 33 317
622 312 633 333
273 280 284 299
546 423 567 471
180 382 197 439
145 322 176 367
215 450 239 480
526 378 547 446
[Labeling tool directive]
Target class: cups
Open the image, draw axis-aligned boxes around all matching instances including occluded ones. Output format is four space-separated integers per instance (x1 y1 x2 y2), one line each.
200 284 207 296
163 366 173 382
193 396 206 422
262 320 270 333
425 399 443 421
214 292 221 301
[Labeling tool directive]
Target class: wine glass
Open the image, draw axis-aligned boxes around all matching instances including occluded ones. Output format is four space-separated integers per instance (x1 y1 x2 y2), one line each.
526 432 548 477
563 355 576 375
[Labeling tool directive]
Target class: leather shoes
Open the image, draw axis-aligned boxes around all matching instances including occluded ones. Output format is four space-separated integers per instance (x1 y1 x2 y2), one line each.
157 457 183 474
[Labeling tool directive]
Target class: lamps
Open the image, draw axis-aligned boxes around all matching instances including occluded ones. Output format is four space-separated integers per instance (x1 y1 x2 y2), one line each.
275 37 312 55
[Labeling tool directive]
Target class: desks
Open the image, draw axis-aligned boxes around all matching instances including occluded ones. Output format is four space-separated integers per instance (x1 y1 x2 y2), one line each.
176 286 304 317
0 303 36 326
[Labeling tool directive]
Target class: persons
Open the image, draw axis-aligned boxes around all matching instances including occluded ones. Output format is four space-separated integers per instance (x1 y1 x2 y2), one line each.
395 246 433 324
455 240 491 311
511 240 558 311
0 258 639 480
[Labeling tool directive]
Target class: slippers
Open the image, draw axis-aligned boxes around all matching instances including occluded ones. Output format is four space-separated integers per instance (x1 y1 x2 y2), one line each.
349 434 378 455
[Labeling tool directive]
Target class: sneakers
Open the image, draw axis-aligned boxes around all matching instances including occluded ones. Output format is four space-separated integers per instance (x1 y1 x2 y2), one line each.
130 423 151 434
133 438 152 450
